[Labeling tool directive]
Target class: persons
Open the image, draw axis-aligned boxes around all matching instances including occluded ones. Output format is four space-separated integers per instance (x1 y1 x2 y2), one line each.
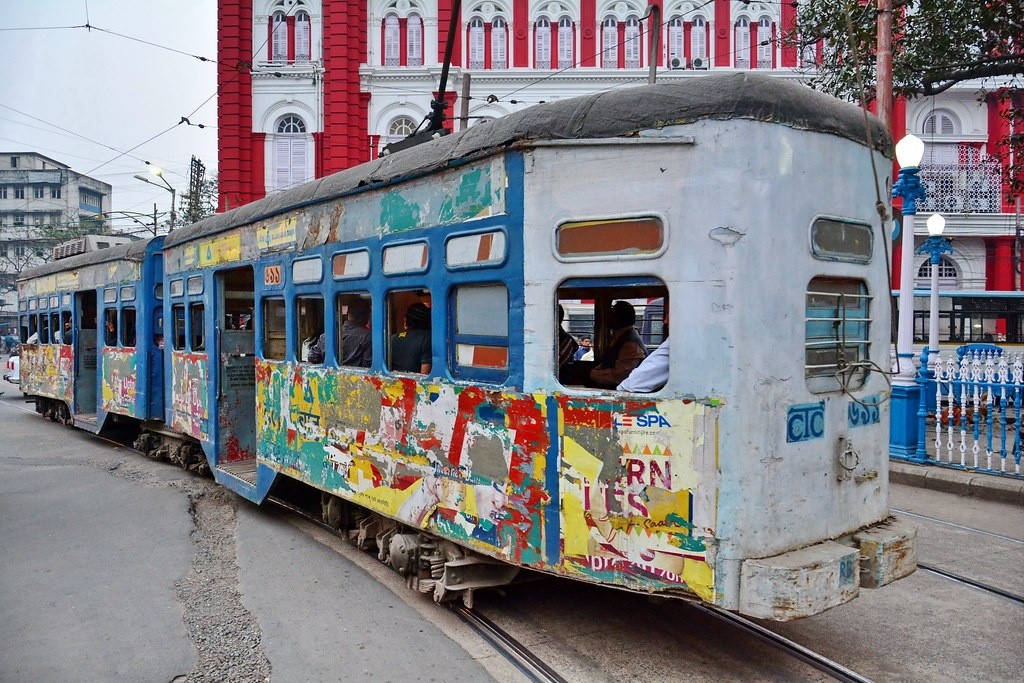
26 315 72 345
106 320 164 348
559 300 669 391
301 299 432 375
5 333 15 354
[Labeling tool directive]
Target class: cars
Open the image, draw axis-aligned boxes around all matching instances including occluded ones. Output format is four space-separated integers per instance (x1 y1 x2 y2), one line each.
3 344 20 383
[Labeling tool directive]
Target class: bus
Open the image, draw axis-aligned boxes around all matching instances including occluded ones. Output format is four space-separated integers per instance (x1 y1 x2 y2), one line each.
17 0 920 614
0 322 18 348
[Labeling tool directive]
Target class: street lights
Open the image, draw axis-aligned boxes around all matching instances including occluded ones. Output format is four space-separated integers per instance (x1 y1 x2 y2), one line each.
889 132 928 461
919 211 955 414
133 169 176 233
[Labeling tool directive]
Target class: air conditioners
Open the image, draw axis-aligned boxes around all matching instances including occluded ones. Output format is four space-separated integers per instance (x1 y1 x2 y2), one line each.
920 178 989 212
671 57 686 70
692 58 709 70
